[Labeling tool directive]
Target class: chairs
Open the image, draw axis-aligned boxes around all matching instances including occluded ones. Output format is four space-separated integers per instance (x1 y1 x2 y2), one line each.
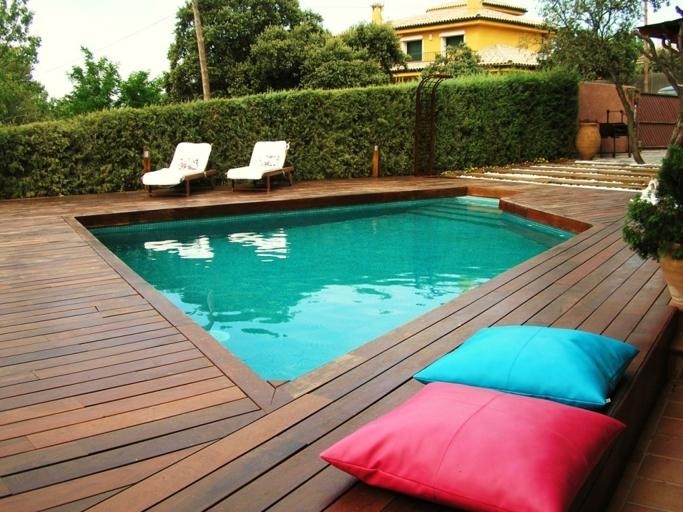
136 138 221 202
227 137 296 200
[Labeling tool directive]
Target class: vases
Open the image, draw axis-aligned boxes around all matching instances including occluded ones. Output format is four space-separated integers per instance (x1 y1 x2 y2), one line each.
575 118 602 163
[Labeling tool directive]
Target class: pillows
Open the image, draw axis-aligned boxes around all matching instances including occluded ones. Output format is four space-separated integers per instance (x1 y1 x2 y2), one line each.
410 324 641 417
315 374 628 511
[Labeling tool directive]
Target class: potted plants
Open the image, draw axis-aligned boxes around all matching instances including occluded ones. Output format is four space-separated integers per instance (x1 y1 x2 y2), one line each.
622 113 683 316
